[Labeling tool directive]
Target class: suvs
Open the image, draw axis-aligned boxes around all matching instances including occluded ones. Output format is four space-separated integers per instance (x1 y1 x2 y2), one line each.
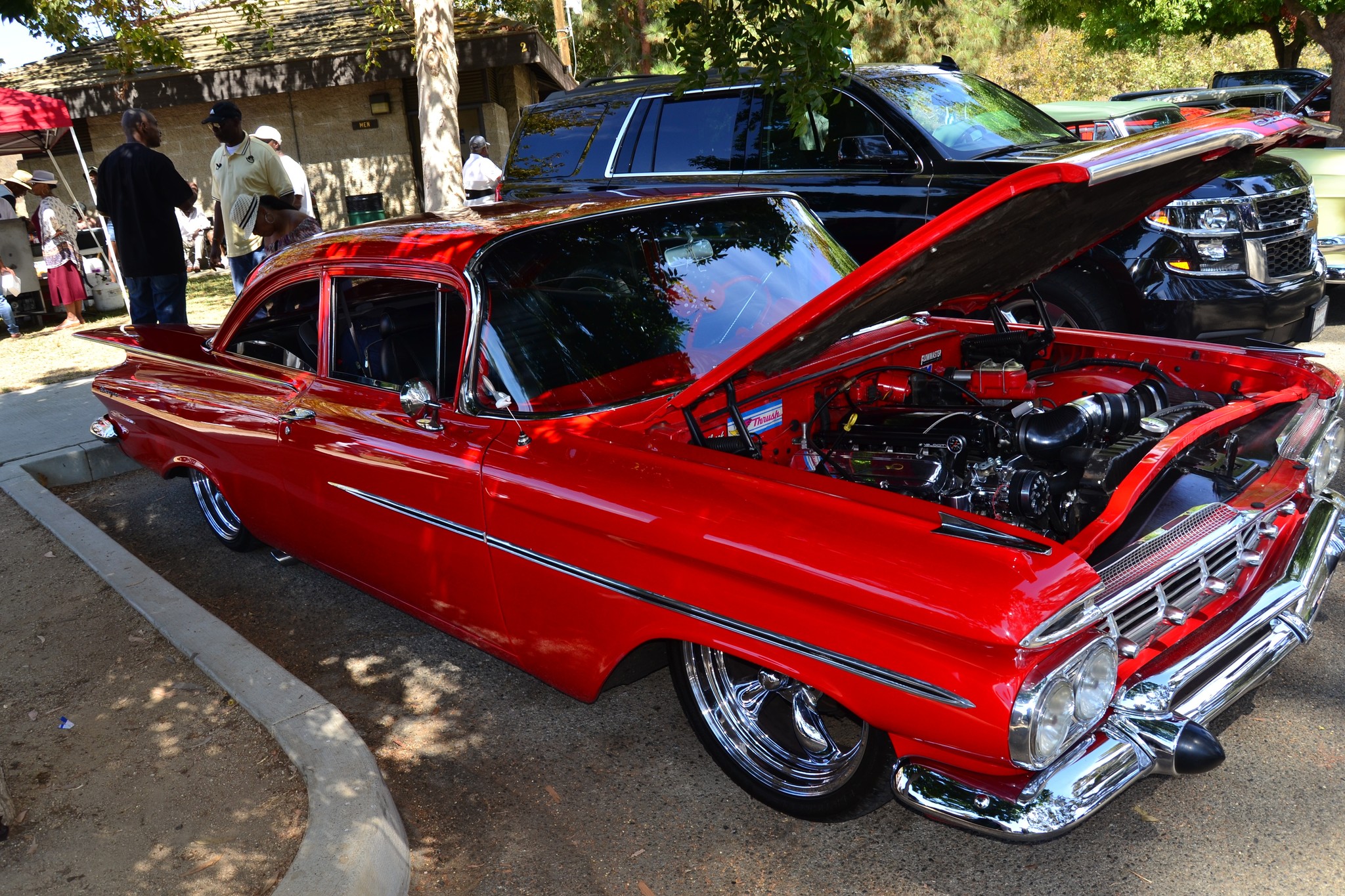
493 63 1329 347
931 67 1345 285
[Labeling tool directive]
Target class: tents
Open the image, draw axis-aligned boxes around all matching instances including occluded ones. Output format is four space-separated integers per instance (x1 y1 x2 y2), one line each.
0 87 132 323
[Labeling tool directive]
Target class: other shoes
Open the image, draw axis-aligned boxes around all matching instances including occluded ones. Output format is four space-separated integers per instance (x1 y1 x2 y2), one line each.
186 265 193 273
54 318 85 330
193 267 201 273
11 332 21 339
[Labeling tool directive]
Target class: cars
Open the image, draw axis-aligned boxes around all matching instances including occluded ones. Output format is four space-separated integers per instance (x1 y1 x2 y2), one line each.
88 110 1344 844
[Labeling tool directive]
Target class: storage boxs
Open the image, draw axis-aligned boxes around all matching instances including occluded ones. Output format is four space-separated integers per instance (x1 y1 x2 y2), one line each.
345 192 385 226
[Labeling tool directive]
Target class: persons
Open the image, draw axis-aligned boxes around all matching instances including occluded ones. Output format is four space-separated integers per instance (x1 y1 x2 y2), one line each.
462 135 502 207
96 108 198 325
0 259 21 339
0 169 39 244
230 193 324 257
248 125 316 219
82 166 119 282
31 170 87 330
67 201 96 230
201 100 295 323
174 206 230 273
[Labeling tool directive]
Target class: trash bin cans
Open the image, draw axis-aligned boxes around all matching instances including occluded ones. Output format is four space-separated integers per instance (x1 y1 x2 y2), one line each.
346 192 386 226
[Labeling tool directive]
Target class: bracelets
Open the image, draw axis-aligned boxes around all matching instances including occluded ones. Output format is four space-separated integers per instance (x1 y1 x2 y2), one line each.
212 238 222 243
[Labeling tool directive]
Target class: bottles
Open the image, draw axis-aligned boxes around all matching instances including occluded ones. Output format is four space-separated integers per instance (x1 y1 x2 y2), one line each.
33 236 40 244
29 235 34 244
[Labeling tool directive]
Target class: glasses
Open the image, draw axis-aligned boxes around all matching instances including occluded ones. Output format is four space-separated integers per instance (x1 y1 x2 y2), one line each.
207 119 231 132
31 182 36 185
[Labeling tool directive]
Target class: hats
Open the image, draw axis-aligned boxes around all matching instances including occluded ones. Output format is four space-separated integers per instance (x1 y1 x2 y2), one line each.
0 170 33 189
249 125 283 147
201 100 242 126
67 202 87 212
26 170 59 185
82 166 99 182
229 193 260 241
469 135 491 149
